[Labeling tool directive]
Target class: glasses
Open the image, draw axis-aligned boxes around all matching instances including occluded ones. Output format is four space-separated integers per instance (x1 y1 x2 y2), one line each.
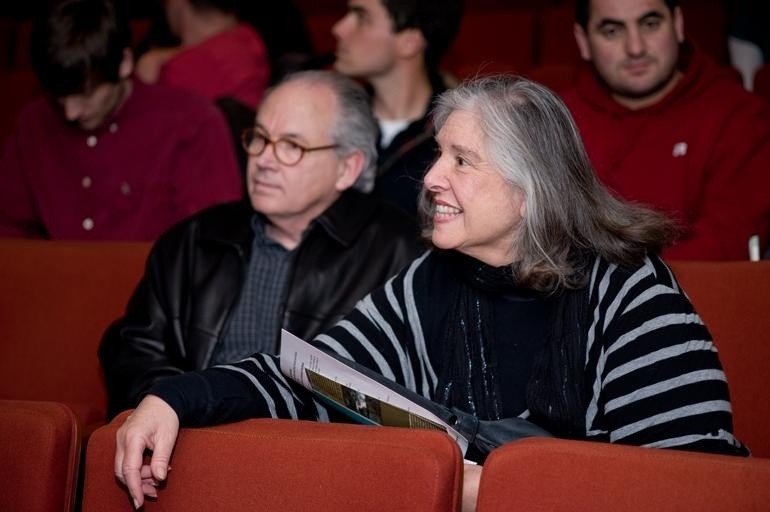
241 126 340 168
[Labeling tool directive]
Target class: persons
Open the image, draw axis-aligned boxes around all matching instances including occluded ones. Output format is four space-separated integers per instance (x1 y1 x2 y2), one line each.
2 11 243 242
98 68 430 424
133 3 272 110
113 69 755 510
218 0 466 232
529 2 769 262
